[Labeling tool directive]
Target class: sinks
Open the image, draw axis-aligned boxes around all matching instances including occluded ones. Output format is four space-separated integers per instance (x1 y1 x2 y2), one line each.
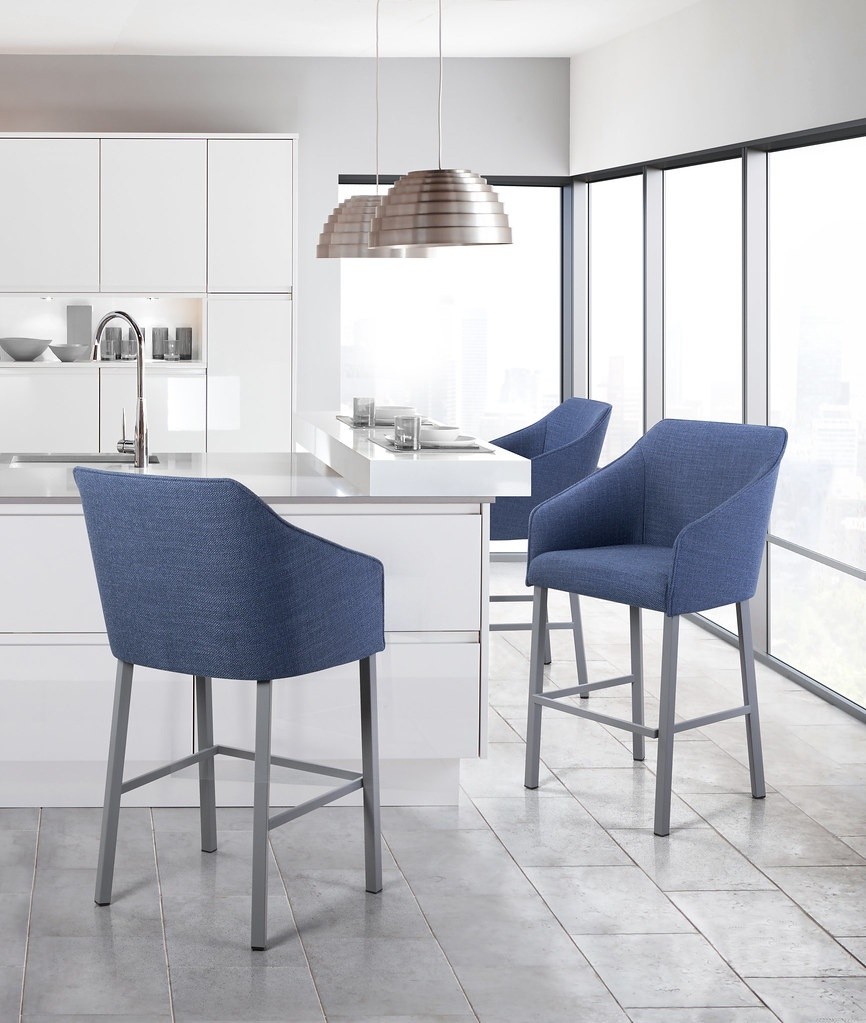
8 454 160 465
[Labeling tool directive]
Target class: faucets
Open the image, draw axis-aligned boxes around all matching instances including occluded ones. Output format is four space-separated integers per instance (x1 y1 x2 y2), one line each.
89 310 150 470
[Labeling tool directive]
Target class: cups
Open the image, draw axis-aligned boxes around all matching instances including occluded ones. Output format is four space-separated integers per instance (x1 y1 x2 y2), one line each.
120 340 138 360
100 340 116 361
105 326 121 359
353 397 375 428
128 327 145 358
176 327 192 360
152 327 168 359
163 340 180 360
394 415 422 450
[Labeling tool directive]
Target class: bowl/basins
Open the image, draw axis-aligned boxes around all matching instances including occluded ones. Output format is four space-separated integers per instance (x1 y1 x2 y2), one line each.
0 338 52 361
421 426 460 441
49 344 89 362
375 406 414 418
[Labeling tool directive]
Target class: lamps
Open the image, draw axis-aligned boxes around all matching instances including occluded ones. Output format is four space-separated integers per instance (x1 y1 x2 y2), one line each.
313 0 432 261
367 0 513 251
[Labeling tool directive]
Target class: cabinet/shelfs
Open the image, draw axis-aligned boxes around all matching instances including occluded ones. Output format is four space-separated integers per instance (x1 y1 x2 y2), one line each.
0 363 206 456
0 498 490 810
0 132 298 294
206 293 297 455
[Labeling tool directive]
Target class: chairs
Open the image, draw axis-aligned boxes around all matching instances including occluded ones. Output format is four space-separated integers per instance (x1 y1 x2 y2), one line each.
486 395 613 701
72 465 385 951
524 418 788 838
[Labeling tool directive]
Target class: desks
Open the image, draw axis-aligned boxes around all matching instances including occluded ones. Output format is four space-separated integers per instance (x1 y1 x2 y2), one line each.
289 402 534 498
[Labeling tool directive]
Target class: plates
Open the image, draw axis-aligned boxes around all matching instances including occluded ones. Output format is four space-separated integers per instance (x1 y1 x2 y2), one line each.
385 434 476 445
375 417 428 424
